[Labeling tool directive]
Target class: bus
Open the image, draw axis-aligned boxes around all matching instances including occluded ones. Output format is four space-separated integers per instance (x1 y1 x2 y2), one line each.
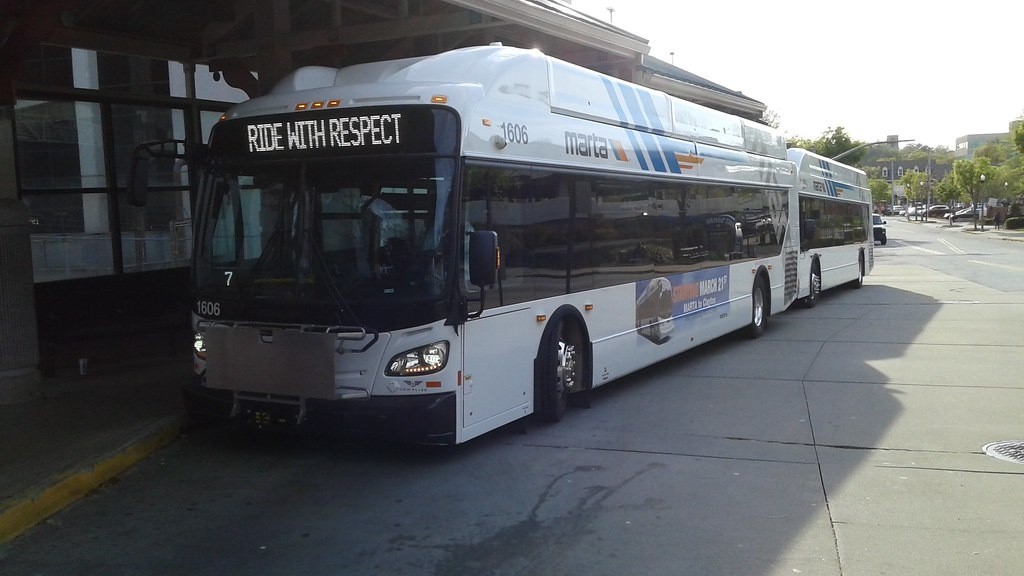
125 42 873 447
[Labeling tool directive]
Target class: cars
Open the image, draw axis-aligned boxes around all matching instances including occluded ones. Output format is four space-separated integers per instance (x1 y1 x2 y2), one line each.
873 213 887 245
883 202 984 220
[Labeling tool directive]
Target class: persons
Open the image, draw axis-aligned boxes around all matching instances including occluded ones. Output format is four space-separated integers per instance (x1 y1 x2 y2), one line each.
874 204 885 216
952 207 956 221
379 200 453 283
994 211 1001 230
